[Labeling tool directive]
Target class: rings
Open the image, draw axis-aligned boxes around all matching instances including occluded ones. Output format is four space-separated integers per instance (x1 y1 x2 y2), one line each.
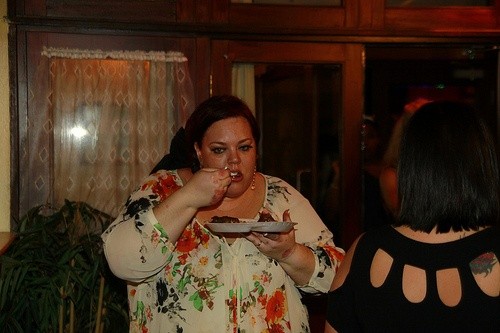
257 240 262 247
263 232 268 236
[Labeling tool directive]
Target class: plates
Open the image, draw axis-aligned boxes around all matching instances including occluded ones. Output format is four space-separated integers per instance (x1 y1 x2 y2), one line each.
203 213 300 234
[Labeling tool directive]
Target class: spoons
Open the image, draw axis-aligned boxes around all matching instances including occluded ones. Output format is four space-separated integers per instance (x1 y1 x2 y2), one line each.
224 166 239 176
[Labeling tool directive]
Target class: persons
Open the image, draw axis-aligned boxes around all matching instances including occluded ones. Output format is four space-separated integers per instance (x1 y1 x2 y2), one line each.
380 99 432 220
323 97 500 333
101 94 347 333
